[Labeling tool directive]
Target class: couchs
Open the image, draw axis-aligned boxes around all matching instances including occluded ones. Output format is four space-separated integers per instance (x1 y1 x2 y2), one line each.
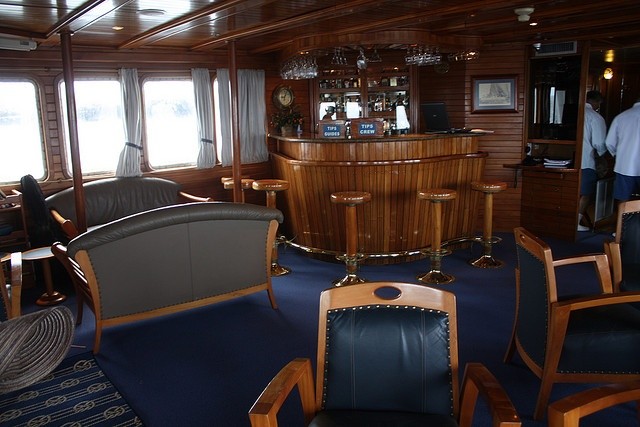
51 202 285 357
44 176 214 240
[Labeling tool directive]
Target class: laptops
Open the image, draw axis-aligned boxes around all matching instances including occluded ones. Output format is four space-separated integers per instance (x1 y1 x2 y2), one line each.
420 102 469 132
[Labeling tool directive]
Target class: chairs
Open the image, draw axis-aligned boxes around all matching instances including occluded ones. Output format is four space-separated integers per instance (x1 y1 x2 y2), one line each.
504 224 639 427
549 383 640 427
248 281 523 427
605 198 639 292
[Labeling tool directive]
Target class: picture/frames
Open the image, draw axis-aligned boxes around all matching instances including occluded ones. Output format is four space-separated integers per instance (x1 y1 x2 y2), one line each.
470 73 519 113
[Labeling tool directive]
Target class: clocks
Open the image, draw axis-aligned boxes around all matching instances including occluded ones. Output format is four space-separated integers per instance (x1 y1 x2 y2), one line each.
272 83 296 112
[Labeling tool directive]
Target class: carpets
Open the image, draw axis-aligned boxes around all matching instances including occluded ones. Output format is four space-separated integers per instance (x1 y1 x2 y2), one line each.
0 351 145 427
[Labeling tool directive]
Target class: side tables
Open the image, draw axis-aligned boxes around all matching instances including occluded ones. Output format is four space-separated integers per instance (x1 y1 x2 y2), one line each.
21 246 67 305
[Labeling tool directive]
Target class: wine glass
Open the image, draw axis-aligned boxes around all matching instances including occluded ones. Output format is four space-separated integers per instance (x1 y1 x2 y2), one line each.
331 48 340 65
356 47 366 70
342 48 348 66
279 53 319 81
404 44 442 66
337 49 343 66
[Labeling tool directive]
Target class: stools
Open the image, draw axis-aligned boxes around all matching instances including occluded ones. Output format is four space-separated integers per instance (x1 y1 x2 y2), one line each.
221 175 250 184
223 178 255 204
252 179 298 276
331 190 373 287
468 177 508 268
416 189 458 284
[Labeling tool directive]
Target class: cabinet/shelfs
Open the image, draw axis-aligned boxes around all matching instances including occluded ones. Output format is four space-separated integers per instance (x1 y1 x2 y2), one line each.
0 189 37 291
312 59 417 134
501 53 591 245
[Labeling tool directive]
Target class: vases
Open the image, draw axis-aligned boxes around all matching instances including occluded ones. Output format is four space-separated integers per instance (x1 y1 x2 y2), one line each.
282 125 293 137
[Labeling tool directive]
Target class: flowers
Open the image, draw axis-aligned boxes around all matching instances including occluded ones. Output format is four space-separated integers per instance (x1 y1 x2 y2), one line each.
271 106 305 129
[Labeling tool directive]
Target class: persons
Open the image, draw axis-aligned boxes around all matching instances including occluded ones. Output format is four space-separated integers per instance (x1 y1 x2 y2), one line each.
576 90 607 233
607 94 640 238
